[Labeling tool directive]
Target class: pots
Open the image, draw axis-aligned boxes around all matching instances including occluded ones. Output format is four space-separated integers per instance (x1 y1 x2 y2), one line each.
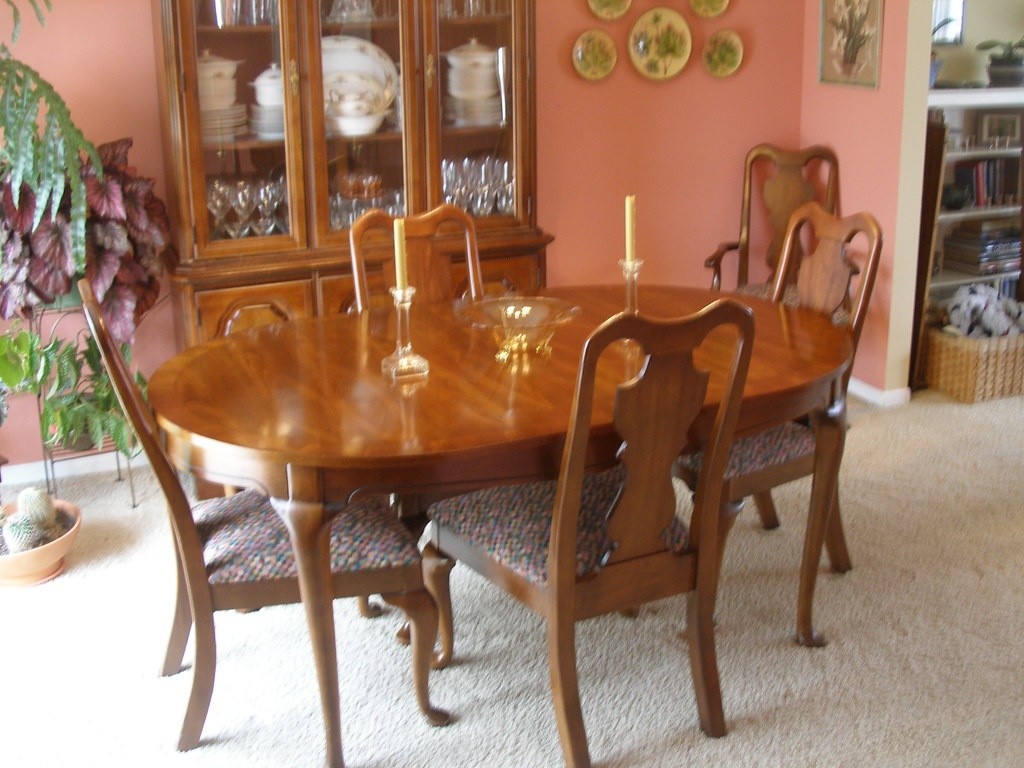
197 48 245 109
246 63 283 105
440 38 497 100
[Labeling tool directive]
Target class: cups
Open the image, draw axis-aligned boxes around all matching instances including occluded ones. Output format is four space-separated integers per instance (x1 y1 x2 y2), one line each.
212 0 486 28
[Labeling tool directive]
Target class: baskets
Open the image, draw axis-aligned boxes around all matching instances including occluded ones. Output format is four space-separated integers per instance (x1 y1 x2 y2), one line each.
918 328 1023 404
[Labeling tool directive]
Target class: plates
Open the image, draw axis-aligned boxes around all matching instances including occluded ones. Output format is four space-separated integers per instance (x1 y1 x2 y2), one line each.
586 0 632 21
572 28 617 80
198 103 286 146
321 34 398 116
701 29 744 77
688 0 730 19
626 5 692 80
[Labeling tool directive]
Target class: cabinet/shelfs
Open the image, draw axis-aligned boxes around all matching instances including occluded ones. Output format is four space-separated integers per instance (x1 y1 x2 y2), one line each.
915 107 1023 389
156 1 550 499
24 307 139 508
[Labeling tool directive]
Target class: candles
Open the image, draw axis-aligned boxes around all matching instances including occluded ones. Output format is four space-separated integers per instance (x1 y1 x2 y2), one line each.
625 192 634 260
392 215 407 295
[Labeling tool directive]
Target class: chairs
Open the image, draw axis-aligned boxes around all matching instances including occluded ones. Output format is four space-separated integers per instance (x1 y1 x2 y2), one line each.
79 142 884 768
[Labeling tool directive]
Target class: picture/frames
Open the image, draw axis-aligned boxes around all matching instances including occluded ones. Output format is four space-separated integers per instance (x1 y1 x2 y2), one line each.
820 0 886 88
928 16 953 90
974 113 1020 146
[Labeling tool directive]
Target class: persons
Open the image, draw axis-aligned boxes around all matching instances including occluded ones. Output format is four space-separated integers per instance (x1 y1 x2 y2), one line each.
943 215 1024 274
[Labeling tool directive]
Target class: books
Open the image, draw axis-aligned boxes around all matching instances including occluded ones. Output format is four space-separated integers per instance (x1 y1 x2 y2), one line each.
953 156 1024 209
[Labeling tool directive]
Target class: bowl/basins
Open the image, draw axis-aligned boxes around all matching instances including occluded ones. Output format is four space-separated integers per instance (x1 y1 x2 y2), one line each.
325 112 392 140
459 294 582 353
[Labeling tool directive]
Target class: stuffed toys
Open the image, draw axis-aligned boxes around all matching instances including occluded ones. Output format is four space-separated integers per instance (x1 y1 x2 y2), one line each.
947 283 1024 339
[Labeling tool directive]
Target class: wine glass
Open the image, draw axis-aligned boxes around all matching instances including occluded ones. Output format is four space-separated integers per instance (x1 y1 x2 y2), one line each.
207 157 517 240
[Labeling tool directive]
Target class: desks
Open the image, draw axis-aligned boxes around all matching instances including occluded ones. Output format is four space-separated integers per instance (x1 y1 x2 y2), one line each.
145 285 859 766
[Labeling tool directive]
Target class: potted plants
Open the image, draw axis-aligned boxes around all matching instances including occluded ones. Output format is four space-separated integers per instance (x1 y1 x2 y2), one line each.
0 329 151 457
975 36 1024 90
0 36 170 340
